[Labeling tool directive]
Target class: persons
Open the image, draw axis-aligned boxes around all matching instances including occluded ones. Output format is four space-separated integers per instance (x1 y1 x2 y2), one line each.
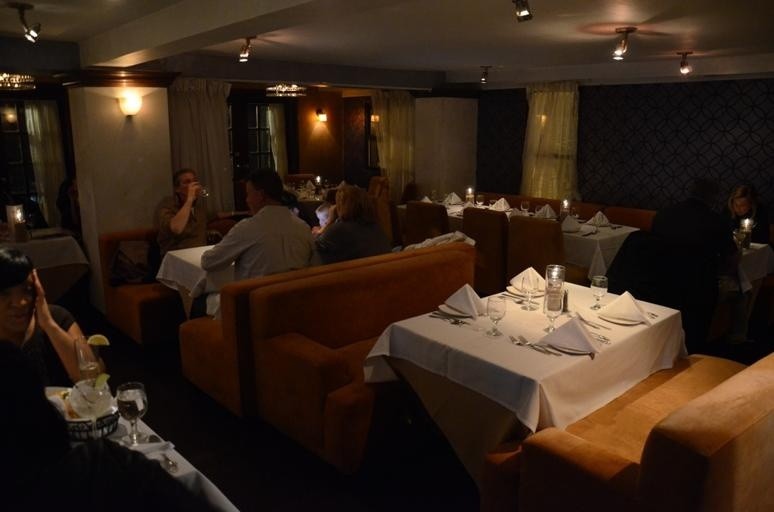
314 203 332 229
716 178 773 251
149 166 211 263
188 166 326 320
313 183 394 265
640 178 741 356
0 246 101 388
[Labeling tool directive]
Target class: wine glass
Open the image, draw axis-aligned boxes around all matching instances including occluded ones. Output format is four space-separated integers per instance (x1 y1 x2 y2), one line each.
195 185 209 199
116 380 150 444
485 296 507 338
588 275 609 310
520 276 538 312
70 381 114 444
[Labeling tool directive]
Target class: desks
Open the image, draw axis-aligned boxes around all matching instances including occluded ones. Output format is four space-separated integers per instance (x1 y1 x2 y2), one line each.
0 226 91 305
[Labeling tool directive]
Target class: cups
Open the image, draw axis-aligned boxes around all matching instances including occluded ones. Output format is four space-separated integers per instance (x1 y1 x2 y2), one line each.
74 335 102 388
542 262 566 333
474 193 544 215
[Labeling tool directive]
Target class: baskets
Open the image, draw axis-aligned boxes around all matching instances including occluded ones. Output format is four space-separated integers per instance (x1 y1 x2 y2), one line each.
64 410 119 443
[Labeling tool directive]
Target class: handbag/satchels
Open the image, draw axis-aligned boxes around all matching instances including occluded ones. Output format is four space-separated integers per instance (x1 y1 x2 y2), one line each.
105 246 150 286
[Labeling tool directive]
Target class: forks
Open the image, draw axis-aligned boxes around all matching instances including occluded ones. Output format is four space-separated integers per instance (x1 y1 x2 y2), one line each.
508 334 562 358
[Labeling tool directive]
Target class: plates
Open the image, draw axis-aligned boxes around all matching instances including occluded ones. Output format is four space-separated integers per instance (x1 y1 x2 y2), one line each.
549 342 592 354
438 303 481 319
600 315 643 327
505 286 545 297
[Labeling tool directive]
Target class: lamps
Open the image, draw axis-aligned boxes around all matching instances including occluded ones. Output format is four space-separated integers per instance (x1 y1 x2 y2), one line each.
1 74 36 92
513 0 533 24
676 52 694 76
239 35 257 63
611 26 637 61
479 65 492 84
266 82 308 98
6 202 24 241
7 4 42 43
